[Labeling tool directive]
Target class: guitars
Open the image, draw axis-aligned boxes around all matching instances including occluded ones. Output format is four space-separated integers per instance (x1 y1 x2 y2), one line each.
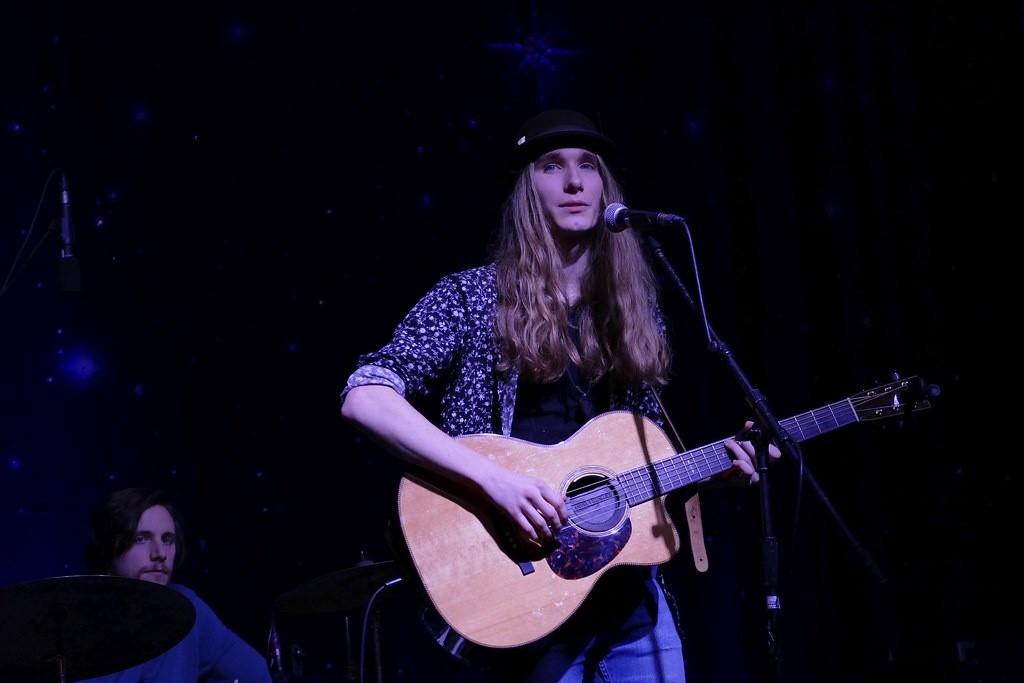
395 370 944 665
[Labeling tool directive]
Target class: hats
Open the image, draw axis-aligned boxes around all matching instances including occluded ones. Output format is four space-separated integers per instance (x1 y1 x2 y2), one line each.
514 108 616 160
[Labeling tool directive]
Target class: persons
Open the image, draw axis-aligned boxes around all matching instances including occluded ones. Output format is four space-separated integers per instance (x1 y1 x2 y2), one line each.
70 487 272 683
339 109 780 683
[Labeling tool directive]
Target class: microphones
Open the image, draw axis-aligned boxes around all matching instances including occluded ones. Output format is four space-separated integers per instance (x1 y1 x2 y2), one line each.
603 202 685 233
62 176 73 256
272 626 283 671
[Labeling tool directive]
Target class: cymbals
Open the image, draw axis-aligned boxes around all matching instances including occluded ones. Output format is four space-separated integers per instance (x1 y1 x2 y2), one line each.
0 574 196 683
280 558 402 614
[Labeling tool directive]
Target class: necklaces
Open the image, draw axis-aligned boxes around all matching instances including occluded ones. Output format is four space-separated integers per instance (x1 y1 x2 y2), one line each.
565 320 592 396
